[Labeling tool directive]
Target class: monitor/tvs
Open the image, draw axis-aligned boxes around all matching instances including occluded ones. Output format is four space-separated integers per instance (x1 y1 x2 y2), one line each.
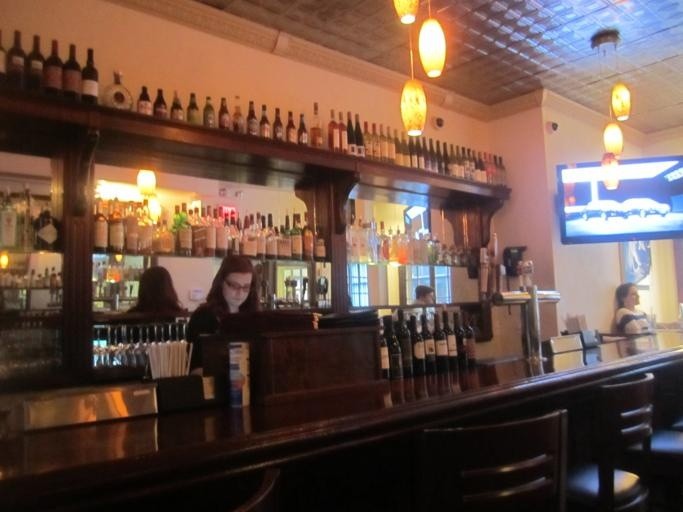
556 155 683 244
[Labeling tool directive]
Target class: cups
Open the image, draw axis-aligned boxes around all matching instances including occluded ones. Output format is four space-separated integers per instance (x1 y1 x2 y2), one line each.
678 304 683 321
647 314 657 334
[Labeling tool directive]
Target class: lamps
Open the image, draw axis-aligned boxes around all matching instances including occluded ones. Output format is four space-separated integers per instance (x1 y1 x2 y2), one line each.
590 30 633 192
391 0 447 140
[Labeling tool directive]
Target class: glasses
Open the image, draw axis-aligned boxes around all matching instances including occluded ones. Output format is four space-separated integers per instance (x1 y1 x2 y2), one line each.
222 279 250 292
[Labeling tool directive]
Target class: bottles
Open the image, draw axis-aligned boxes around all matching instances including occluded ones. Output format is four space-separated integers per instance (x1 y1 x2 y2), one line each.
44 38 63 95
273 107 284 141
170 91 185 121
355 113 366 157
260 103 272 138
0 265 63 288
340 110 348 154
203 96 216 127
345 111 357 155
103 67 134 111
412 312 426 377
153 88 168 120
460 147 469 179
362 120 374 160
391 129 404 165
35 202 61 250
385 125 395 161
138 85 153 117
408 136 418 168
328 108 340 151
453 310 470 370
93 198 326 260
498 157 507 185
63 42 82 100
401 321 414 378
16 183 36 250
435 140 445 174
370 123 381 160
8 27 28 89
416 136 425 169
378 319 390 379
247 99 259 136
489 153 497 185
482 152 491 184
219 98 231 130
493 155 501 185
232 96 245 132
390 334 403 380
442 141 452 176
432 311 450 376
454 145 465 178
26 34 45 90
0 31 8 85
424 310 437 377
450 144 458 176
0 186 20 250
399 131 412 167
422 137 431 170
187 92 202 123
311 102 324 148
91 262 144 282
347 216 469 266
442 309 460 371
298 112 308 146
379 124 388 161
82 48 100 104
472 150 481 182
427 137 438 171
477 151 488 183
462 311 477 370
466 148 476 180
287 110 297 143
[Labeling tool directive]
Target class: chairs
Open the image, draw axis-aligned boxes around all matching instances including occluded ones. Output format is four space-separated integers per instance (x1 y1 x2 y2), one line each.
423 409 566 509
233 463 292 511
568 370 658 509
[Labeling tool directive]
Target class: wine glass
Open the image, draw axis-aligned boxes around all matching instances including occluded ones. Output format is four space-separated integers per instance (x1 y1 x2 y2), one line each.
2 310 62 380
92 322 189 367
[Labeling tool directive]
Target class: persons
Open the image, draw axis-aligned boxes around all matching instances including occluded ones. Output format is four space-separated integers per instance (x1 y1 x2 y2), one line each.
185 255 263 345
126 266 187 316
413 285 433 304
614 284 651 335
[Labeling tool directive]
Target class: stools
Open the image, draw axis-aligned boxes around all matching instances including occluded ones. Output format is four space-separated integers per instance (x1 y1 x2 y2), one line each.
625 425 683 512
